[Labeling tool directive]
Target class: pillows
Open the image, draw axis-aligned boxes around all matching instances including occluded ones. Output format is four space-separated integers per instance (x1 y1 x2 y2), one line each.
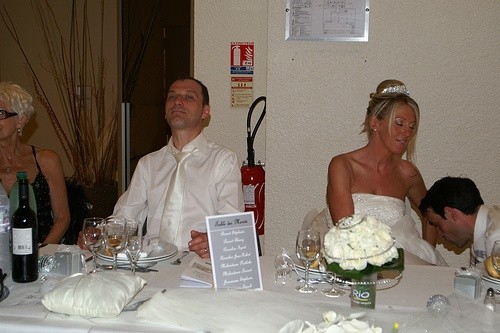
40 272 147 319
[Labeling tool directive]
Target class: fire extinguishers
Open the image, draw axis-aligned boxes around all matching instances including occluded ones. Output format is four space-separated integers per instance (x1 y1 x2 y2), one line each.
240 150 266 235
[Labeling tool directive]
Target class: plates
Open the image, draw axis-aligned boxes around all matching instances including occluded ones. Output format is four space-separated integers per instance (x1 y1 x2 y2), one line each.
94 238 179 269
476 263 500 284
290 254 327 277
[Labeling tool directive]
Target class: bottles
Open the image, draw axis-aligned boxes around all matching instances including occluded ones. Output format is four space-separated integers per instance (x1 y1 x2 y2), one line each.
0 178 11 281
10 179 39 284
323 272 346 298
483 288 496 311
5 171 38 253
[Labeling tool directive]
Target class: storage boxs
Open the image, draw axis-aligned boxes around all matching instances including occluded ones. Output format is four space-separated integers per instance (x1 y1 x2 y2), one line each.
455 275 482 299
55 252 84 276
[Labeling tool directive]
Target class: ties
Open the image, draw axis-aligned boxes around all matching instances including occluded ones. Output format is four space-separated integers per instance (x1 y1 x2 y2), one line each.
159 148 199 245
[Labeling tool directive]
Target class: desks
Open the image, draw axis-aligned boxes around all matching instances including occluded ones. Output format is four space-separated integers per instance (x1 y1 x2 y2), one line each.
0 242 499 332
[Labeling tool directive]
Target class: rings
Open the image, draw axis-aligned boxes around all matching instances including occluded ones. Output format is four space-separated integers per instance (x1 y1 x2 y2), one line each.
205 248 208 253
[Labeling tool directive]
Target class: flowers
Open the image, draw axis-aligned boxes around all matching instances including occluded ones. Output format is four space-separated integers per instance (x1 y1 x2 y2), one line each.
320 215 403 278
278 310 383 333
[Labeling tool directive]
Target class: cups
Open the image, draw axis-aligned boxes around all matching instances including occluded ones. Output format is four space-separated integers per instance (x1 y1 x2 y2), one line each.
492 240 500 278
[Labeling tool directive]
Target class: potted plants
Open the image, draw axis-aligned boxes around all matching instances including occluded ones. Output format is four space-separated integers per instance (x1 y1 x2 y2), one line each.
0 0 157 232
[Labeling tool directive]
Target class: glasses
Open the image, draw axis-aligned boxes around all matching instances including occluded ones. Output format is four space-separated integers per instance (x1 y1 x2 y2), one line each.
0 110 17 119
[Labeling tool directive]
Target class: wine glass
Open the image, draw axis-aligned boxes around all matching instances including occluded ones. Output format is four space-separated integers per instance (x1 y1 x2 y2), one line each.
295 229 321 293
82 218 143 276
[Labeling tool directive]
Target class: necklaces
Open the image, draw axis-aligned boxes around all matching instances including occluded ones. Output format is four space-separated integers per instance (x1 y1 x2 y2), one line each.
1 167 16 174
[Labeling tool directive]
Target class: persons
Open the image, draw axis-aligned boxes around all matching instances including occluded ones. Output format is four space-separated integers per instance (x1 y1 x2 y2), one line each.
0 80 71 245
79 77 245 259
310 79 449 268
418 177 500 267
